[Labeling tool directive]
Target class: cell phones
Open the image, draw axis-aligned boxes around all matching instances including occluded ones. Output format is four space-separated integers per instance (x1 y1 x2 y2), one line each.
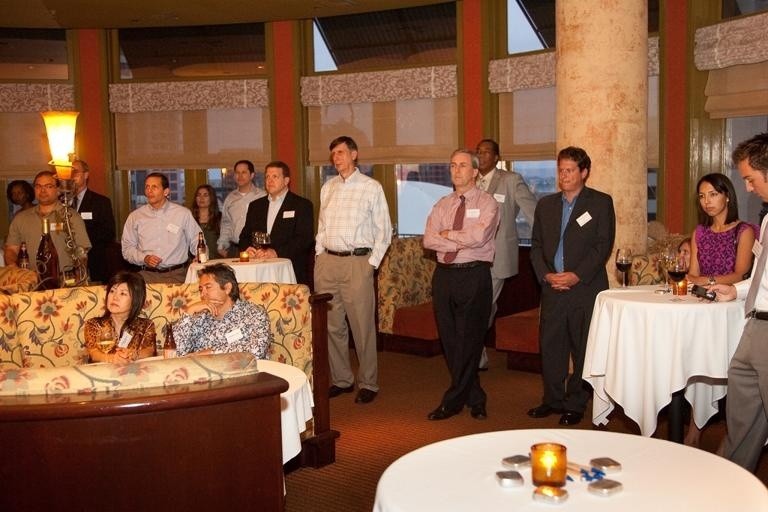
692 283 716 301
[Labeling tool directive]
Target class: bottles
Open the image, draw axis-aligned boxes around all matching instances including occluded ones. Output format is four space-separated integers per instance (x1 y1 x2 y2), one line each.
197 231 206 263
18 241 29 269
34 217 60 289
163 323 177 360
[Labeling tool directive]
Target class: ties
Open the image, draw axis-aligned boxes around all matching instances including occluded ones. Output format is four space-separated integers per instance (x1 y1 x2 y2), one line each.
69 196 79 211
478 178 485 189
443 194 467 264
743 221 768 315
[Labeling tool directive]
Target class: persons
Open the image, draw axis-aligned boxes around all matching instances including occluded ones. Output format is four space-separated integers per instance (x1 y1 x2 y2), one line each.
5 160 116 289
187 161 315 289
678 237 693 269
314 136 394 405
524 146 615 429
121 173 209 285
469 140 541 372
421 150 504 420
693 172 755 287
82 271 157 363
708 131 767 474
174 262 270 360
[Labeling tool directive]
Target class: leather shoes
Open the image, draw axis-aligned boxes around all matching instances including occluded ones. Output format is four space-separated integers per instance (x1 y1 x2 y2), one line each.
527 404 563 417
427 404 464 420
329 384 354 397
471 402 487 419
559 411 584 425
355 387 377 404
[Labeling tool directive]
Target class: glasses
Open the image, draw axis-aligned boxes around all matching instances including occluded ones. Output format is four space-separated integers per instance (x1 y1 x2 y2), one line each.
195 192 209 198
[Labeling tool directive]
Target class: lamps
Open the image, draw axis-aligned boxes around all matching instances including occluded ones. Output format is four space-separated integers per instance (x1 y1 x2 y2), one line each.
35 108 93 289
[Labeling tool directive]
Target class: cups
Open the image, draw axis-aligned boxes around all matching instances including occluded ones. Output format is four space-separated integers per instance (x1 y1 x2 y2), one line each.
672 280 688 295
240 251 249 262
529 441 567 487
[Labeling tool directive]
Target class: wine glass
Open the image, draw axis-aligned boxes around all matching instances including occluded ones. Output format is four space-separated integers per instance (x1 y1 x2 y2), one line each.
659 244 674 292
666 256 687 303
615 247 634 290
96 326 116 370
252 231 272 264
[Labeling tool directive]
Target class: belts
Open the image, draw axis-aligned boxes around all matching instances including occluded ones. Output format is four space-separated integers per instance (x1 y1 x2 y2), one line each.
142 260 187 273
324 247 367 256
744 309 768 321
437 261 479 270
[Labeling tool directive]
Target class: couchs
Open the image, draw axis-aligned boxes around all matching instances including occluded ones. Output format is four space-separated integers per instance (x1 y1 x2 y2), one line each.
493 303 547 374
0 262 39 296
1 351 289 510
0 282 339 474
368 230 445 357
